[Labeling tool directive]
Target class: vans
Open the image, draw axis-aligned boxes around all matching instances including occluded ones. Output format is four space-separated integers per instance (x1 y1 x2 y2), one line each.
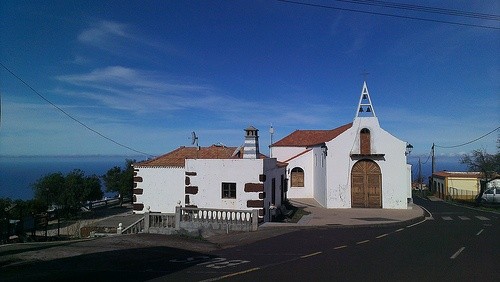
476 187 500 205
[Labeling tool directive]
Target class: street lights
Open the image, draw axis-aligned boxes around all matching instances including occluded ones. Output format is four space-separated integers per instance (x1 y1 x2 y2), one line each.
270 124 273 158
